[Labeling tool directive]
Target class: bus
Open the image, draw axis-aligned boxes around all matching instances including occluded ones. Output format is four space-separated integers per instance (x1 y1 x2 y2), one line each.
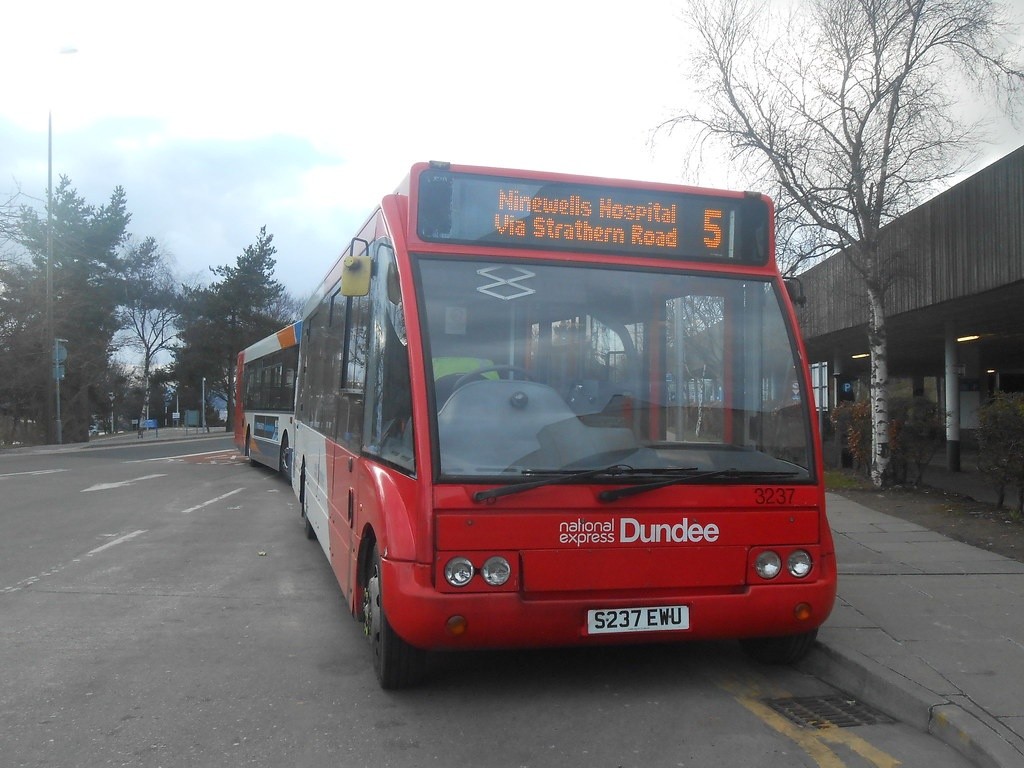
290 157 839 688
234 318 303 486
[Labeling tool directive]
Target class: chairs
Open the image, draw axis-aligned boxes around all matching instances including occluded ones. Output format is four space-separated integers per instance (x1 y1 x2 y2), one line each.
245 384 295 412
302 356 633 453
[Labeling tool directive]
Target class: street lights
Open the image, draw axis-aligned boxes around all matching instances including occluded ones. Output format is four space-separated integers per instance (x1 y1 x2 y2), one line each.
55 339 69 447
